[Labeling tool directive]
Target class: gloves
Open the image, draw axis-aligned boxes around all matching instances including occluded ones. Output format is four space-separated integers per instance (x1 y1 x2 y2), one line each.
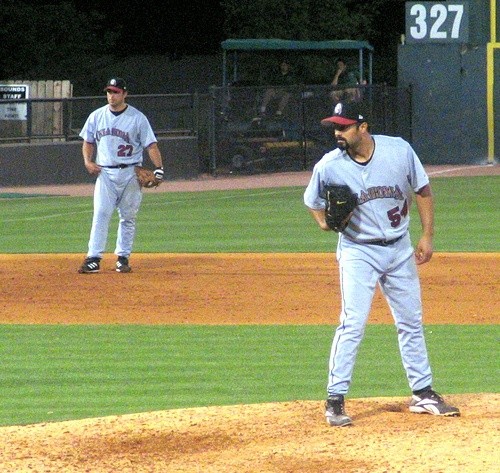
324 184 358 234
144 167 165 188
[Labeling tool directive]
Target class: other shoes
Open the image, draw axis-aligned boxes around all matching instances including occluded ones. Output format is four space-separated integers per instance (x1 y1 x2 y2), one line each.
252 112 266 117
276 113 285 117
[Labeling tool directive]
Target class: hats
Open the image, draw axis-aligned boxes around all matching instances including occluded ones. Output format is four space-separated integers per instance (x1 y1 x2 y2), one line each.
278 59 290 64
320 102 369 128
101 76 126 93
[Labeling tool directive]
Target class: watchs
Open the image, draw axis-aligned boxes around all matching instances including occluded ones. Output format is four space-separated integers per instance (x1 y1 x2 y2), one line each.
156 166 164 170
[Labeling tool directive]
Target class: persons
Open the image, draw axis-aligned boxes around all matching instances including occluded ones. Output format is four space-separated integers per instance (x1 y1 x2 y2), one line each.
77 77 164 274
303 100 460 426
329 58 367 105
260 58 297 118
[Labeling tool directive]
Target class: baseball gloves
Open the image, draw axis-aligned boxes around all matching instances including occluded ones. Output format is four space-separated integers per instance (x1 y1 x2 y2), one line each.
136 166 158 187
324 184 356 236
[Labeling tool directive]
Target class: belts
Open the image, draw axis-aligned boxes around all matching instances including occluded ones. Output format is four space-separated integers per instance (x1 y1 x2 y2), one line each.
362 235 402 247
101 162 138 169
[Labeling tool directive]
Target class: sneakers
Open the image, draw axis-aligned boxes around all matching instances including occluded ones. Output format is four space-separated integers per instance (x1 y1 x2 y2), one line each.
77 257 101 274
409 391 460 416
324 397 354 428
114 255 130 272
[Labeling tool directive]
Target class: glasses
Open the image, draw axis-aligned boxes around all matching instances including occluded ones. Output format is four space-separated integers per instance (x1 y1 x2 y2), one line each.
328 123 357 132
280 64 288 67
